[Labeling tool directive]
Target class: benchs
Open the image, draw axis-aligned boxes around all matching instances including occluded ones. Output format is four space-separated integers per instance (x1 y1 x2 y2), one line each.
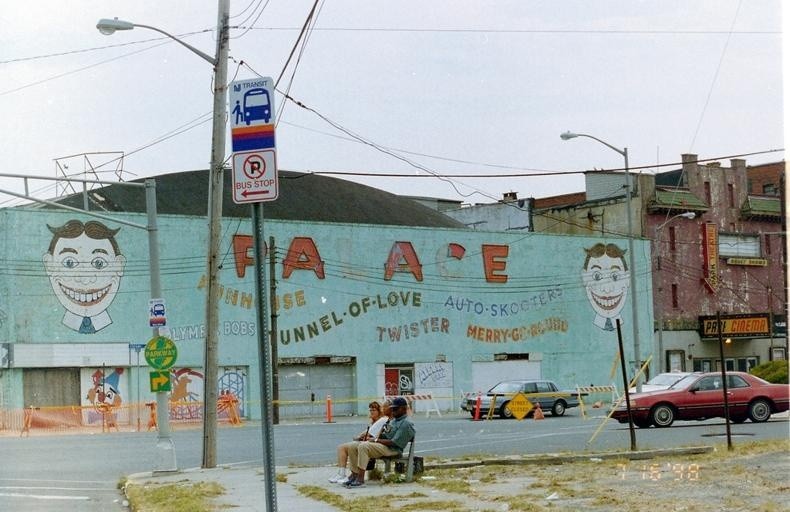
381 430 416 480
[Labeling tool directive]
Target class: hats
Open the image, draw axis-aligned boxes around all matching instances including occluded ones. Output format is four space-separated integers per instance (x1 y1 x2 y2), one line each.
389 398 407 407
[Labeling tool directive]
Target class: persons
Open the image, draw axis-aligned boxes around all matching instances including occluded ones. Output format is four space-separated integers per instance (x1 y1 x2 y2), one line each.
337 398 394 484
329 400 383 483
346 398 415 486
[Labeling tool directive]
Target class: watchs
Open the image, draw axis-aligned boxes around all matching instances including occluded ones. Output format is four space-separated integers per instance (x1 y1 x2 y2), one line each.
374 438 378 443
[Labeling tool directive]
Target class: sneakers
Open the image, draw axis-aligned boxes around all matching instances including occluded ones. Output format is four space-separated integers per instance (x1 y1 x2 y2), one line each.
330 474 364 485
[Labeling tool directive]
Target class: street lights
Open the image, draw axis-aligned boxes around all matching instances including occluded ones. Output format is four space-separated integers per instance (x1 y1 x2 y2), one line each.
560 129 643 395
95 16 229 469
653 211 696 373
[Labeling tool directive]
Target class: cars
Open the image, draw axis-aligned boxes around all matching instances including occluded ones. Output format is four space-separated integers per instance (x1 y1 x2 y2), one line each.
622 369 693 396
605 371 790 428
460 380 583 417
